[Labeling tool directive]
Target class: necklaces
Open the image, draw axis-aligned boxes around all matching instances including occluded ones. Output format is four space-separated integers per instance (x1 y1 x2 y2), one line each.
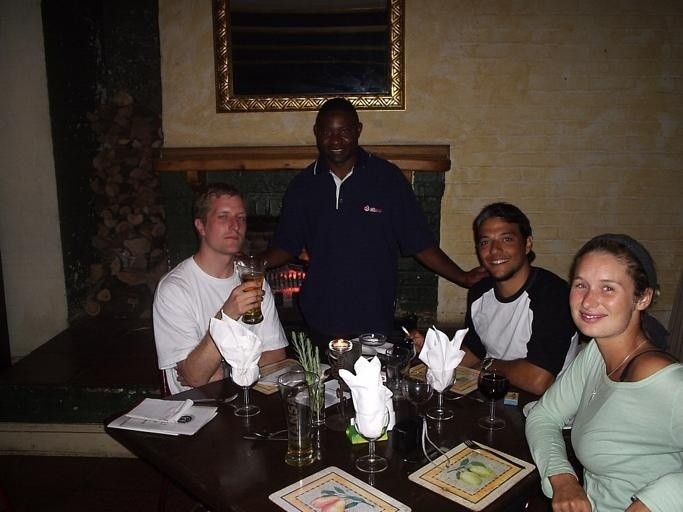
587 339 648 407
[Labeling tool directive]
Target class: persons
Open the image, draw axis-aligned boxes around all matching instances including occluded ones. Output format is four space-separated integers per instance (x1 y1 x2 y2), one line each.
234 98 490 349
151 181 290 395
524 232 683 512
404 201 571 397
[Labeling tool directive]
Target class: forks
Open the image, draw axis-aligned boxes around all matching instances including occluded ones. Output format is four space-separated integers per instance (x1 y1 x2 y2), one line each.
188 392 239 404
465 440 526 470
254 429 288 438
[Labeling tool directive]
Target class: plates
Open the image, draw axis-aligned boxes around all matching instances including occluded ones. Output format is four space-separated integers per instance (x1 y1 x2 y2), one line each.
523 400 574 429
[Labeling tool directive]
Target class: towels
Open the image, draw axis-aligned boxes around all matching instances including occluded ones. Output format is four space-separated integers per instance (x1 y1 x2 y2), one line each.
336 323 469 438
207 309 264 387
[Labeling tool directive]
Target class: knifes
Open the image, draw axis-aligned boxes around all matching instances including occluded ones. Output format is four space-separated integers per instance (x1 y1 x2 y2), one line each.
242 436 289 441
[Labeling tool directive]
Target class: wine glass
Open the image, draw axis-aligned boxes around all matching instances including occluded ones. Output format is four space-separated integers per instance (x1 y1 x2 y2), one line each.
230 358 260 417
328 333 511 474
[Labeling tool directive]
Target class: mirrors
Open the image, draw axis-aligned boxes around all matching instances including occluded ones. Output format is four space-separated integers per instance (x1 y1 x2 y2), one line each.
209 2 408 113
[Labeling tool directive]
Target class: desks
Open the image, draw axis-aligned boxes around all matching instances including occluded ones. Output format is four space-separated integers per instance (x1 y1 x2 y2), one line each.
102 331 536 512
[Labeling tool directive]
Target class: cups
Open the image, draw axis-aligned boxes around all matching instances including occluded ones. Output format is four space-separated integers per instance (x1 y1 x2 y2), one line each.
277 370 320 467
235 260 267 324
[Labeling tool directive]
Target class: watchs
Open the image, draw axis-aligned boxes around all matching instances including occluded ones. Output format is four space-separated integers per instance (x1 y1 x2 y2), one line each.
479 357 498 371
